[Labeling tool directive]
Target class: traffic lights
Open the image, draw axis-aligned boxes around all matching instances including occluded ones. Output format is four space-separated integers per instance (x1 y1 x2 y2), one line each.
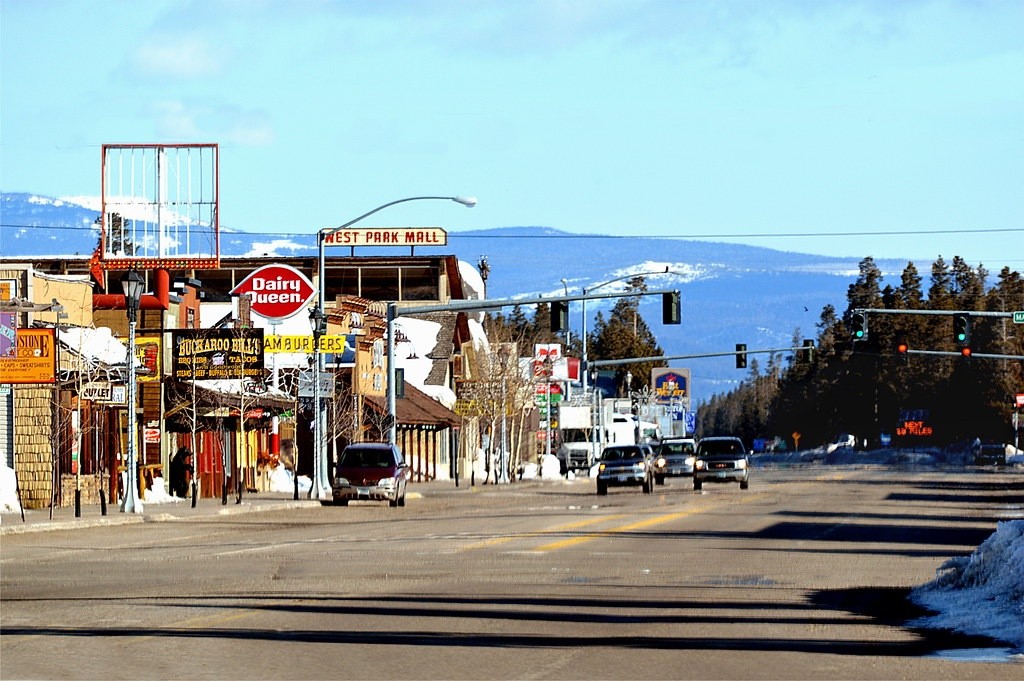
896 343 908 366
853 313 868 340
954 313 969 343
962 346 971 368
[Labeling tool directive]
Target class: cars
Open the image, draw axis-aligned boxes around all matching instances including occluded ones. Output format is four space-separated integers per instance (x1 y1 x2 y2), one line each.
595 442 661 495
653 439 698 485
694 436 750 491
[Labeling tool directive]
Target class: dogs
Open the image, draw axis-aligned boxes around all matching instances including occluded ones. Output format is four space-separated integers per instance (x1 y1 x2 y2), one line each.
169 446 194 497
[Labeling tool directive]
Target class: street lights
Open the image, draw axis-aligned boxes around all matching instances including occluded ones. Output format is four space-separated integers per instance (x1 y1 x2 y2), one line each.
590 365 598 465
561 279 570 352
119 263 145 514
543 355 553 456
319 196 477 492
497 344 510 485
308 301 325 499
583 270 688 396
625 371 633 398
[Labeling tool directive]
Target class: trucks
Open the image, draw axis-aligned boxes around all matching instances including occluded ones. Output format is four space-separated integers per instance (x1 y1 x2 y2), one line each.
556 406 609 477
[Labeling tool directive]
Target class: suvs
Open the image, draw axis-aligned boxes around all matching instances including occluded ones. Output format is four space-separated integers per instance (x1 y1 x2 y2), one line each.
330 441 408 506
